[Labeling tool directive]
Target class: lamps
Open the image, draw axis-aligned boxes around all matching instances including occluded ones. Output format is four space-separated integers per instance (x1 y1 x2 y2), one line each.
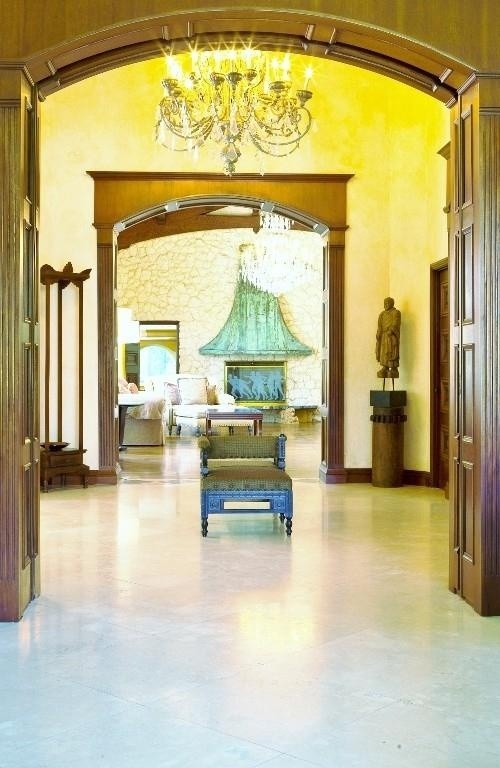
236 207 316 298
154 42 315 182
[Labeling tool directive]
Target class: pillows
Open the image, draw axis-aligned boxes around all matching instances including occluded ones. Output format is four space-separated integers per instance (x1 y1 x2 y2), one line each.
167 376 217 405
118 377 139 394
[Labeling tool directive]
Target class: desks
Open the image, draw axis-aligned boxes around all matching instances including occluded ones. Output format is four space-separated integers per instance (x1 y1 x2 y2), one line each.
118 400 144 451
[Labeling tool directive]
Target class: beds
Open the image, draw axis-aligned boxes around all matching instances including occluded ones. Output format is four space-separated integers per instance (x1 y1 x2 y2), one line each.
162 394 253 436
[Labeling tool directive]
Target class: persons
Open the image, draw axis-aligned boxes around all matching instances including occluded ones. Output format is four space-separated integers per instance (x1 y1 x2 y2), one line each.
375 296 401 378
226 370 286 401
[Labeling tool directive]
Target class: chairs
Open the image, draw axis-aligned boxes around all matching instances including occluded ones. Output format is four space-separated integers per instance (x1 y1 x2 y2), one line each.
197 432 294 537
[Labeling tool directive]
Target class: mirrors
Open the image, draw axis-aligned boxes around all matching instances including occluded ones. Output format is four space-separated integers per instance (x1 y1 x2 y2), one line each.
139 320 180 391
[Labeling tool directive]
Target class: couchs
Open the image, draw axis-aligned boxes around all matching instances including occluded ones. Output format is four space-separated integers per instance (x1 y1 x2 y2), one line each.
117 392 167 447
